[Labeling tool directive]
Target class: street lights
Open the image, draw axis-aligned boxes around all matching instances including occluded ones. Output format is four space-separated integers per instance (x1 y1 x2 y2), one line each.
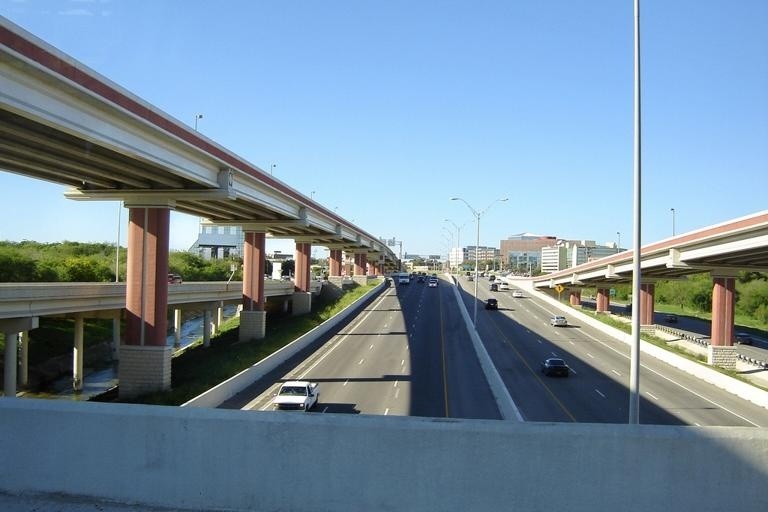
441 197 508 330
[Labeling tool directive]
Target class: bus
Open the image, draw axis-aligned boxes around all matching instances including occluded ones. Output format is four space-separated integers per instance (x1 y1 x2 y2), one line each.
398 273 410 284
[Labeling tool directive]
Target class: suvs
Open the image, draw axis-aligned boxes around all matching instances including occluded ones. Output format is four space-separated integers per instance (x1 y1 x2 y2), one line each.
485 298 498 310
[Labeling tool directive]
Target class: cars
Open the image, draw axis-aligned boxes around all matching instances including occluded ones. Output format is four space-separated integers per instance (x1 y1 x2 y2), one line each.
466 271 495 281
543 358 569 377
551 315 567 327
664 314 678 323
410 271 439 288
487 279 510 291
513 290 522 298
734 333 751 345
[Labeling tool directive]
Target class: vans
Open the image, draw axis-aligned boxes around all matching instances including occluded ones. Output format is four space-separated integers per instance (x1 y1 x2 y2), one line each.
169 273 183 285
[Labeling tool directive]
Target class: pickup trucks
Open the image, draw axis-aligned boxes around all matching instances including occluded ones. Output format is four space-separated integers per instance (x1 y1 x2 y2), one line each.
273 381 320 413
